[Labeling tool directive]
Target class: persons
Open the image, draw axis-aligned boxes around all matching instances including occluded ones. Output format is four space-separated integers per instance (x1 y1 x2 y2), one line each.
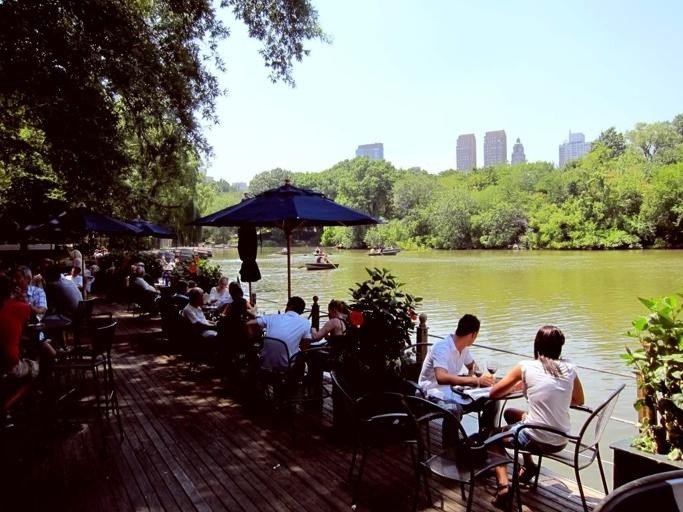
412 313 500 460
0 239 395 429
489 325 584 506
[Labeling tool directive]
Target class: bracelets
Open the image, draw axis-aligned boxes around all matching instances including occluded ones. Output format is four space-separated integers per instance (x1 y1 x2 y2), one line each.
513 386 516 393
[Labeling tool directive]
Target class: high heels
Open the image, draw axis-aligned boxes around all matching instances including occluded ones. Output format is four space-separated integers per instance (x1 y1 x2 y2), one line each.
491 485 512 508
519 463 537 483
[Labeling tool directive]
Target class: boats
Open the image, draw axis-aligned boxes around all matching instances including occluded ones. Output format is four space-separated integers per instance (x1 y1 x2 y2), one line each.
368 253 383 256
305 263 339 270
281 247 287 255
334 244 345 249
383 247 396 256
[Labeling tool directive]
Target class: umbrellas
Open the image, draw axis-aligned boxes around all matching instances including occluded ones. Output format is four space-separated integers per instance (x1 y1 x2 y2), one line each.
121 213 177 239
235 192 262 308
182 178 388 301
7 203 177 301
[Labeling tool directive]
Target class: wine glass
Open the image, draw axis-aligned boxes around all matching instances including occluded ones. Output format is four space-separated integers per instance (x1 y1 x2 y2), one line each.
34 309 44 326
486 360 498 387
472 364 484 389
